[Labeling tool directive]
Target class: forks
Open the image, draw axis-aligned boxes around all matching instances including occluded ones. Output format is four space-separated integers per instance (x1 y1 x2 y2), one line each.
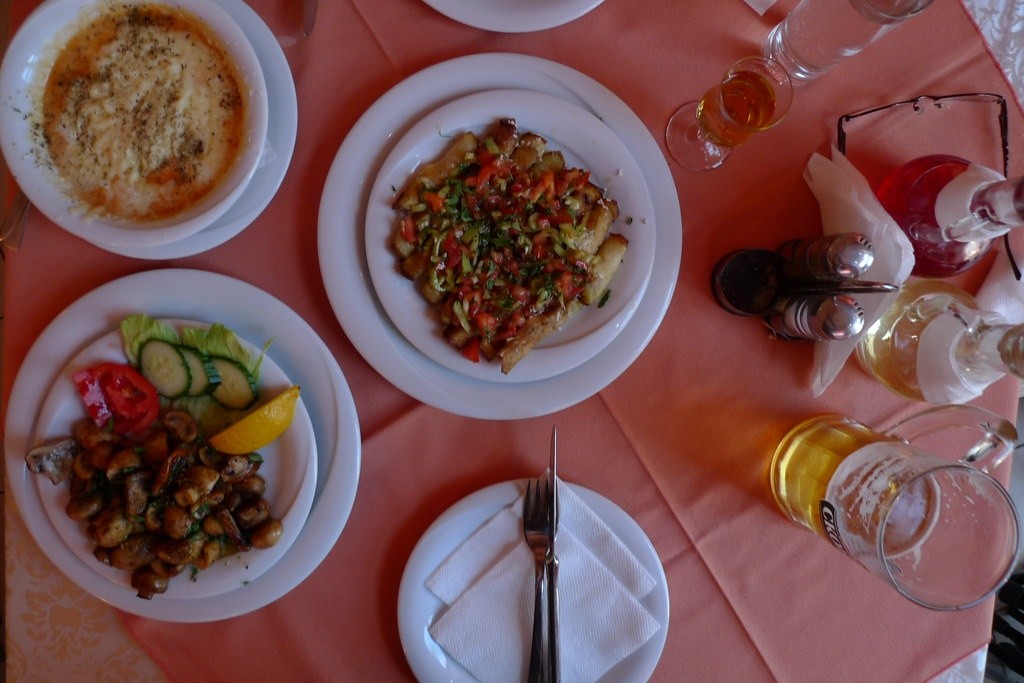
524 476 560 683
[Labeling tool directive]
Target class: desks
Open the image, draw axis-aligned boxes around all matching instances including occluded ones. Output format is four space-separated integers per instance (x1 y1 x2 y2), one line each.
1 0 1024 682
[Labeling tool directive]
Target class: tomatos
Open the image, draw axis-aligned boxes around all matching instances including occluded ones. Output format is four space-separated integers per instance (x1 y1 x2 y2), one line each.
399 150 584 358
72 364 158 438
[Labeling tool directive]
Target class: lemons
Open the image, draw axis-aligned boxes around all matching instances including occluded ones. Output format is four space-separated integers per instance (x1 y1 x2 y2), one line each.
208 385 300 455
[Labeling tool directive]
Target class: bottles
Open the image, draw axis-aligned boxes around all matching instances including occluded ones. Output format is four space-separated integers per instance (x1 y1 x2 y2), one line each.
782 232 871 286
876 154 1024 279
855 280 1024 406
757 293 865 341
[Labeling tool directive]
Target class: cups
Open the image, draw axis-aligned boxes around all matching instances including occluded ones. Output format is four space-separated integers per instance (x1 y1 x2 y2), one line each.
764 0 933 85
769 406 1024 612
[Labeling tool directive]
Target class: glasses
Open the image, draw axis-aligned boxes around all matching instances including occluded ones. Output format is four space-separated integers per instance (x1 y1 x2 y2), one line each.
836 91 1022 282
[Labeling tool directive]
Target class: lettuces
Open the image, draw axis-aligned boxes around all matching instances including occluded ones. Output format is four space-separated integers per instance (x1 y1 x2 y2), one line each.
119 314 278 443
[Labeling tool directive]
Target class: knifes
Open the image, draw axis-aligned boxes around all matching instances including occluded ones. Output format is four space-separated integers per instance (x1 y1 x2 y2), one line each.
550 425 559 683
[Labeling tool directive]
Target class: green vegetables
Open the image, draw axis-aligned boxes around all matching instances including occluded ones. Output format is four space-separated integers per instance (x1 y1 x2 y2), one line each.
415 158 611 343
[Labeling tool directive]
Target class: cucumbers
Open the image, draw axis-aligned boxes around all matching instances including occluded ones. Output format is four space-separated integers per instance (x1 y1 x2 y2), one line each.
208 355 259 411
138 339 193 400
176 343 223 397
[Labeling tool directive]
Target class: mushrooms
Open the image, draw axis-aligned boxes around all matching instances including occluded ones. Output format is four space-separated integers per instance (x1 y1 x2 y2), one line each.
23 411 282 599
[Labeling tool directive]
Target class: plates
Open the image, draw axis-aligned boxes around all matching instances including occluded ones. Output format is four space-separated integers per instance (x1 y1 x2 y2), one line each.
421 0 606 34
317 51 681 420
364 90 655 384
4 269 361 624
0 2 268 248
398 478 670 683
29 319 317 602
0 2 299 259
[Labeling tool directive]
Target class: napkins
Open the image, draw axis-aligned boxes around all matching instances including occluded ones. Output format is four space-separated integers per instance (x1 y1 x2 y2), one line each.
424 468 661 683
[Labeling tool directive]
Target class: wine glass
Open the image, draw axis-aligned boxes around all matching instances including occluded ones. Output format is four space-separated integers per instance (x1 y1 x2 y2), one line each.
664 56 795 171
